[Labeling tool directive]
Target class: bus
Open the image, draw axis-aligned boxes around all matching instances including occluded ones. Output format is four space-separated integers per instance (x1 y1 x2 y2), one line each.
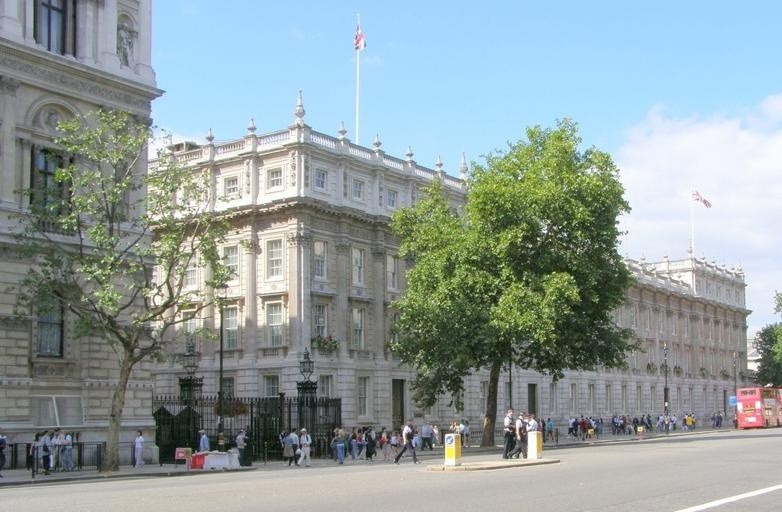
736 384 782 429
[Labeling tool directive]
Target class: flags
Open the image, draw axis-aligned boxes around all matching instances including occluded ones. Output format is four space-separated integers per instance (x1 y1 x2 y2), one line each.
352 24 368 52
691 190 712 209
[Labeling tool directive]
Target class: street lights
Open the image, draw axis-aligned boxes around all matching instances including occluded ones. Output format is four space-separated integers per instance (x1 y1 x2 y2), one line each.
295 346 319 460
178 339 206 455
663 341 672 438
732 350 739 430
216 279 228 451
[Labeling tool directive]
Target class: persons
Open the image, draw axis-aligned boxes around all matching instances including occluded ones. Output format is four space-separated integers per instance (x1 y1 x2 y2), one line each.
329 416 441 465
463 419 472 447
285 427 300 465
279 428 288 461
610 409 723 437
501 406 603 461
197 428 210 452
29 426 76 475
134 430 147 468
458 419 465 447
234 429 248 467
297 427 312 467
449 420 458 432
1 429 7 478
